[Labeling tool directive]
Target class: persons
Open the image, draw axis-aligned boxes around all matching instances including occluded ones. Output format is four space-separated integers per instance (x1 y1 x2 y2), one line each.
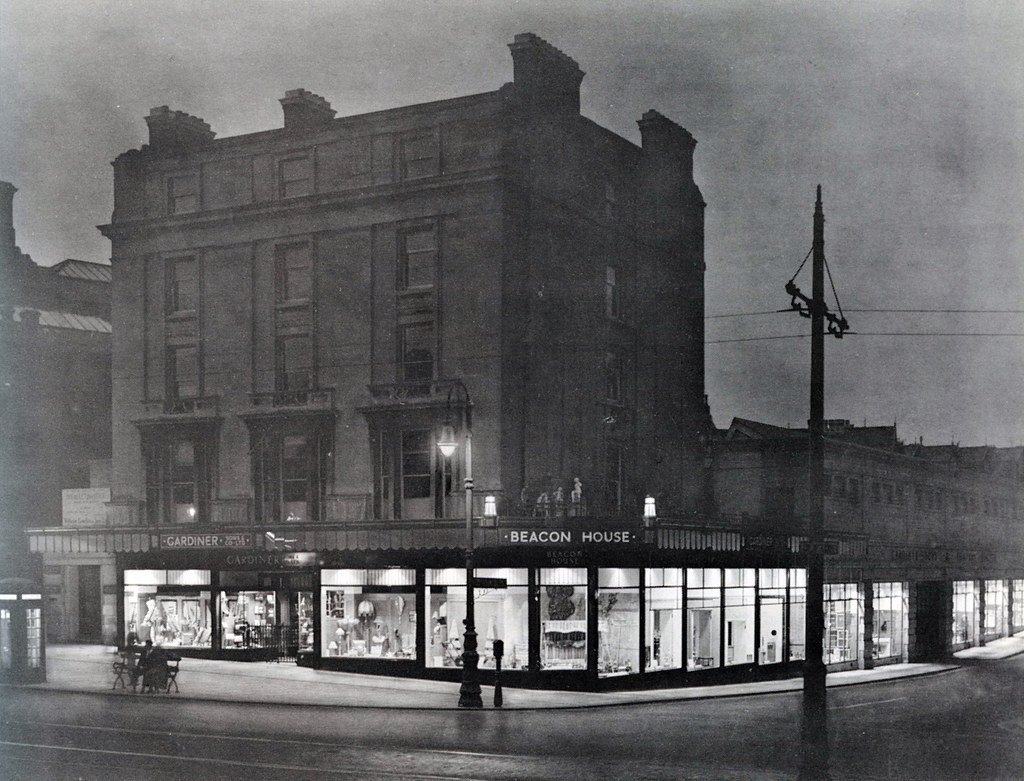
130 640 168 694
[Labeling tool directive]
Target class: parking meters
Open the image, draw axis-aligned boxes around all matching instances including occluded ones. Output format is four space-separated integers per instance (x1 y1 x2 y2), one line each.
493 640 504 707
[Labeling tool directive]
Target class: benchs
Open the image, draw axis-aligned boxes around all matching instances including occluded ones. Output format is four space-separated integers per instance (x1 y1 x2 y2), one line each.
111 654 182 694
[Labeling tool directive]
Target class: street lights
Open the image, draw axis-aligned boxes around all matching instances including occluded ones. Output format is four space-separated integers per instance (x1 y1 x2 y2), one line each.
436 381 483 706
